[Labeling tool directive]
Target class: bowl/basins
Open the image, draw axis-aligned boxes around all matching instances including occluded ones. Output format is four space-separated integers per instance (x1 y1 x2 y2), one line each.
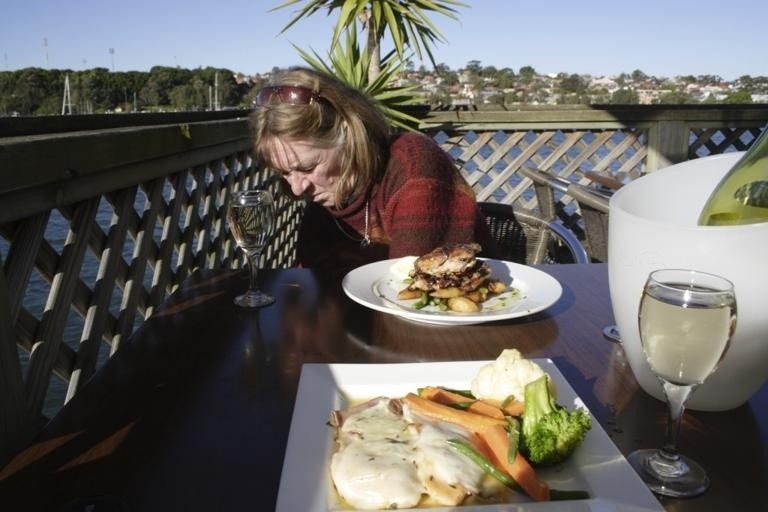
607 149 768 412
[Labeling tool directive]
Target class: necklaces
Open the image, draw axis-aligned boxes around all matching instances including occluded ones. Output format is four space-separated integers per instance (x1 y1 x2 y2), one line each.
322 200 375 252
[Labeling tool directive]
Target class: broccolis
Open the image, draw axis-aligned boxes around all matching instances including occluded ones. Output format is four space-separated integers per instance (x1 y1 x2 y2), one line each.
518 374 593 464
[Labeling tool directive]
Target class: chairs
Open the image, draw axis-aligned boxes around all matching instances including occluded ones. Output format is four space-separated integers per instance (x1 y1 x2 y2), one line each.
476 166 613 264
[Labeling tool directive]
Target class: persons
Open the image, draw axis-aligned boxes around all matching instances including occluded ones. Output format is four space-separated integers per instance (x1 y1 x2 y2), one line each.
252 65 492 268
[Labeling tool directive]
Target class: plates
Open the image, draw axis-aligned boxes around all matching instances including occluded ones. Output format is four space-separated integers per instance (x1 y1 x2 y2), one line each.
342 254 565 328
273 357 668 512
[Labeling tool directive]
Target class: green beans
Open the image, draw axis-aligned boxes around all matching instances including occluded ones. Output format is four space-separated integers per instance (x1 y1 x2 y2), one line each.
446 440 590 500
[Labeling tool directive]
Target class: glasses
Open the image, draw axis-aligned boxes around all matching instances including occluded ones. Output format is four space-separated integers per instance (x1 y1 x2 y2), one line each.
256 85 321 105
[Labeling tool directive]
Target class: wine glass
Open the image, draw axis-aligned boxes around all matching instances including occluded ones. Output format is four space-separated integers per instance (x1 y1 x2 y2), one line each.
226 187 278 308
624 267 738 500
